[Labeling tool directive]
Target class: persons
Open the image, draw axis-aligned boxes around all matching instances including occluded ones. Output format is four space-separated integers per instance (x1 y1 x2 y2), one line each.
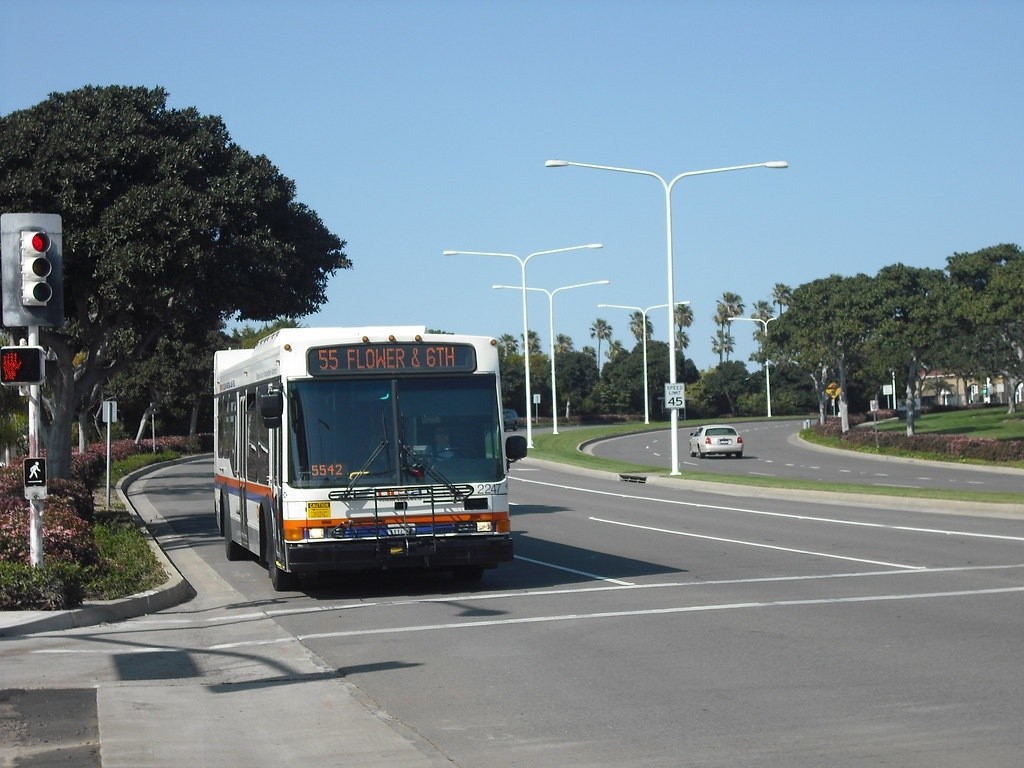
423 424 459 464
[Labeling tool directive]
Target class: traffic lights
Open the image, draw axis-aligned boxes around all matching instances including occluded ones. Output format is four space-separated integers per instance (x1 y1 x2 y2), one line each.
1 213 64 327
0 346 46 386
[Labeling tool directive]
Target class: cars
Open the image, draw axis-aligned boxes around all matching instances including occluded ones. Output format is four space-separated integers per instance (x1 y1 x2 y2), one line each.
504 410 519 432
689 424 744 459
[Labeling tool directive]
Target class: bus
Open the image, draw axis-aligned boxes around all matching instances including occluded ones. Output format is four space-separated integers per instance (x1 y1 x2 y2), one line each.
214 325 528 592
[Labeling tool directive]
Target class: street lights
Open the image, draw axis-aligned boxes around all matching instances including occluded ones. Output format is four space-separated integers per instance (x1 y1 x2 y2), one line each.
493 280 610 434
443 244 604 449
598 301 691 425
546 160 788 477
726 318 778 418
